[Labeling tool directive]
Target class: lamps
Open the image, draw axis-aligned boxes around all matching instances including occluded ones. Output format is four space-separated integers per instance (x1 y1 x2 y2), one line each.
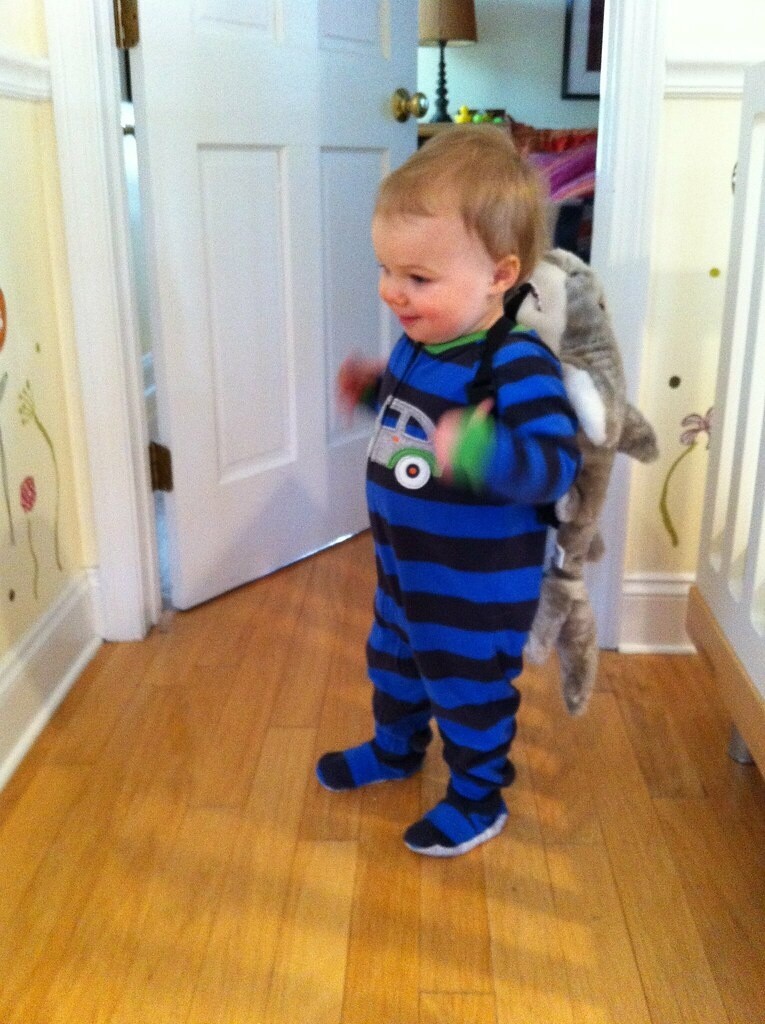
416 0 481 122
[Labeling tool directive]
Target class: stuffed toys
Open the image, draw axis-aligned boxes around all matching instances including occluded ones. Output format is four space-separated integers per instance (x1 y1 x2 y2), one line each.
516 249 659 716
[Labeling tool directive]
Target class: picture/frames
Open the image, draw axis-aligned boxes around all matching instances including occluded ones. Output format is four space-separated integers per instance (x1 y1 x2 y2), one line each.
559 0 606 101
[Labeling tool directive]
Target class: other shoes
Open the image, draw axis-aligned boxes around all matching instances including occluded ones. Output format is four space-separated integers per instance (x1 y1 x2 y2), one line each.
401 782 510 856
315 734 418 788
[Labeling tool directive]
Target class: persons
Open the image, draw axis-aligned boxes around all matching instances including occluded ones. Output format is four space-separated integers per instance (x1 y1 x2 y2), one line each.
317 126 584 856
